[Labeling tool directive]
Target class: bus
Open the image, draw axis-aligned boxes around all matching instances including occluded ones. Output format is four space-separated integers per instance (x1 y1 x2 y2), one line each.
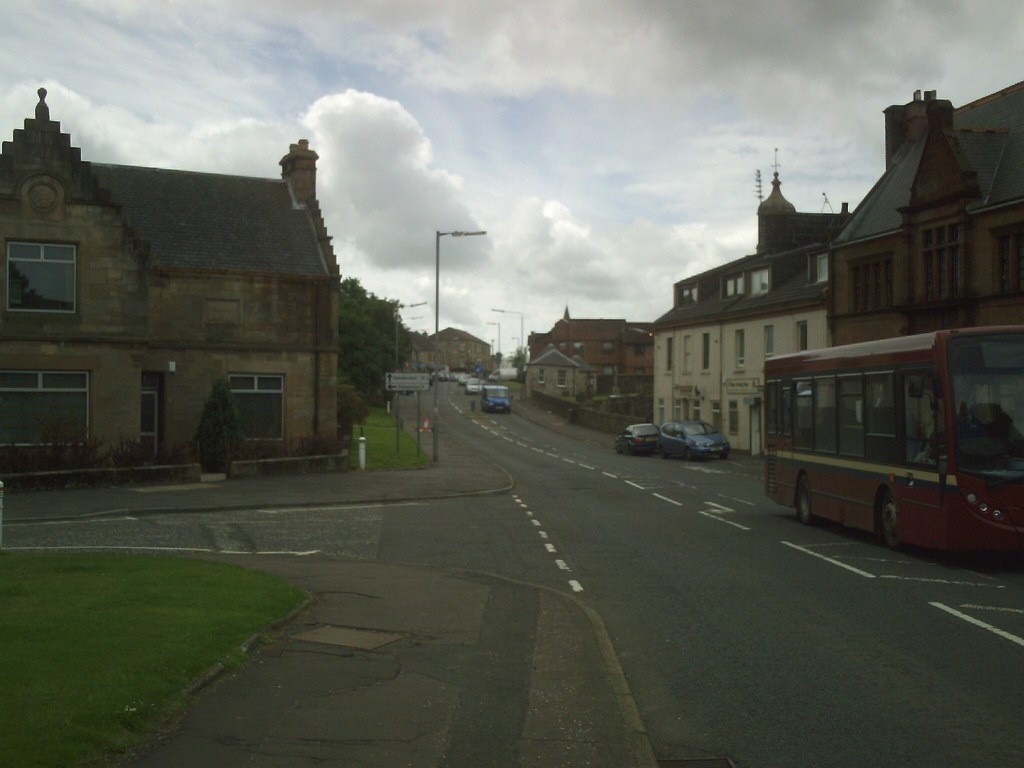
766 325 1023 553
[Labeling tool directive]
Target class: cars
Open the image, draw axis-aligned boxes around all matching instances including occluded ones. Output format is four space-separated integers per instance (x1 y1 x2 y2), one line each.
658 419 731 462
459 375 468 385
616 423 658 456
466 378 482 393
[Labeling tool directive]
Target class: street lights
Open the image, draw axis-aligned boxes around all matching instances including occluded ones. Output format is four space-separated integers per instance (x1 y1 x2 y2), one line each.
493 310 524 348
487 321 500 353
432 231 487 462
395 301 426 371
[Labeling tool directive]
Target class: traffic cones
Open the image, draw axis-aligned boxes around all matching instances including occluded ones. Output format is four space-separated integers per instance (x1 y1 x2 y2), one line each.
420 416 432 432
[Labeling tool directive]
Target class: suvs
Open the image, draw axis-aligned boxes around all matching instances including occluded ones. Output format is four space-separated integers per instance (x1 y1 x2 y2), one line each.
481 385 514 414
